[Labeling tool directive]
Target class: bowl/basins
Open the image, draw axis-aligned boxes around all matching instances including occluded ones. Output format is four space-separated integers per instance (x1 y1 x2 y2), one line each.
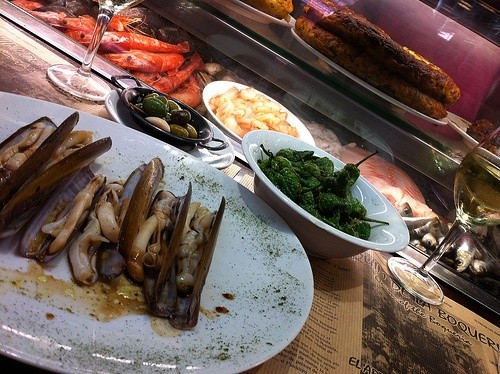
242 130 410 259
112 75 229 151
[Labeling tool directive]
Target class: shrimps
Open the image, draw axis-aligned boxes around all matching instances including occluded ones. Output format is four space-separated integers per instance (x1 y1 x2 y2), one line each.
11 0 207 109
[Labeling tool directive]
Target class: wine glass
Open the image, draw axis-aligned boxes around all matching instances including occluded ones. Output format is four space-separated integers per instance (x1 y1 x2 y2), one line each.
47 0 143 101
387 126 500 305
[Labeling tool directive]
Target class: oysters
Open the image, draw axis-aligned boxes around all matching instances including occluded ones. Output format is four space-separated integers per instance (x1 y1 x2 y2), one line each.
0 111 226 331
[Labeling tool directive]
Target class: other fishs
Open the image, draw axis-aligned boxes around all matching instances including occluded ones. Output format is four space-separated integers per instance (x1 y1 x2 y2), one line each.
398 202 500 278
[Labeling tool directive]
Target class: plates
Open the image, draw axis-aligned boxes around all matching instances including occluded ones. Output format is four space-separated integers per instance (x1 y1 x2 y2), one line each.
290 27 449 126
204 0 296 27
202 81 314 141
105 88 235 169
447 108 500 165
1 90 314 374
207 111 249 164
